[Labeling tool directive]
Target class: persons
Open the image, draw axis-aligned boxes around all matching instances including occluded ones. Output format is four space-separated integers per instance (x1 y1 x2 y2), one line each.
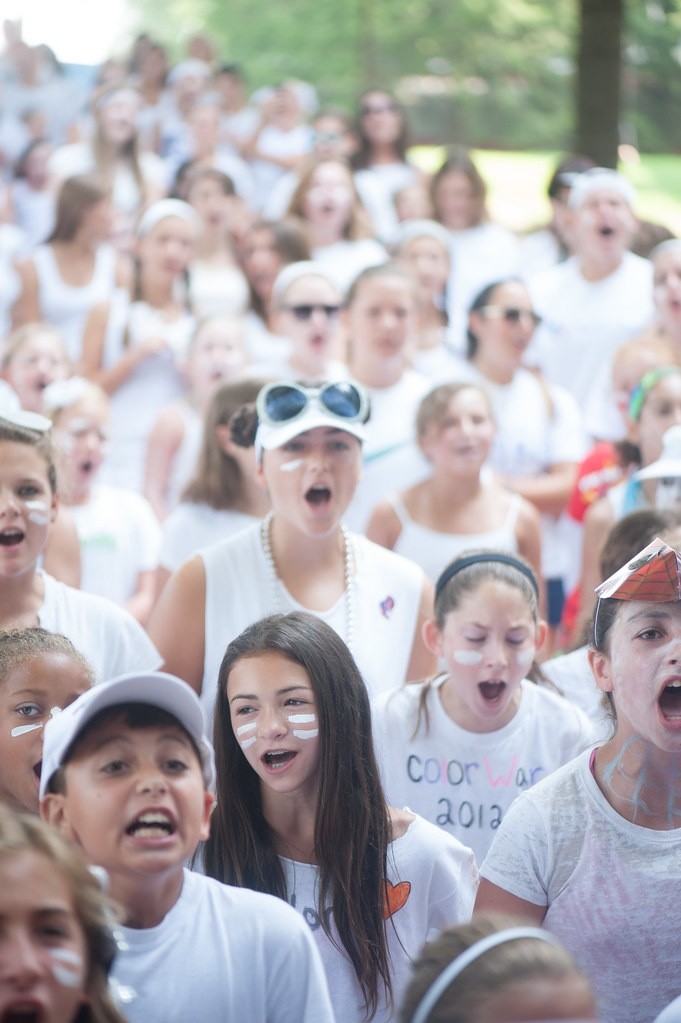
0 15 681 1022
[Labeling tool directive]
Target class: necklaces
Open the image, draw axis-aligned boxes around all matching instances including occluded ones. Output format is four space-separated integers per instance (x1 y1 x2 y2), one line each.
262 510 354 666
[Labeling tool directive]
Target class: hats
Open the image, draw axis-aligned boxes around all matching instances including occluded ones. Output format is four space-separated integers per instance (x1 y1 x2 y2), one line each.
39 669 213 800
256 387 365 465
635 426 681 481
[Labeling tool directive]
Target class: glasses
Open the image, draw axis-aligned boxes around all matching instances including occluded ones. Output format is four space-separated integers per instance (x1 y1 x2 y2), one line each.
3 408 53 440
282 305 339 319
477 305 544 328
257 384 371 429
67 416 112 444
